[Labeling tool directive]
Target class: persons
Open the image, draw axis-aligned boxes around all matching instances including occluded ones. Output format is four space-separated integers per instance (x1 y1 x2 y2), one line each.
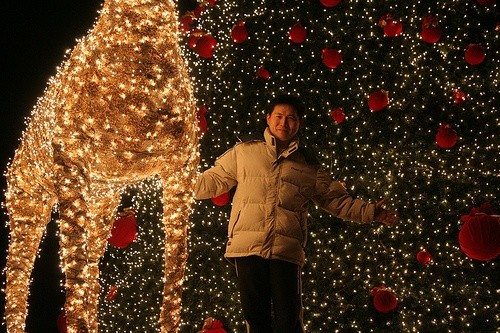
192 95 401 333
